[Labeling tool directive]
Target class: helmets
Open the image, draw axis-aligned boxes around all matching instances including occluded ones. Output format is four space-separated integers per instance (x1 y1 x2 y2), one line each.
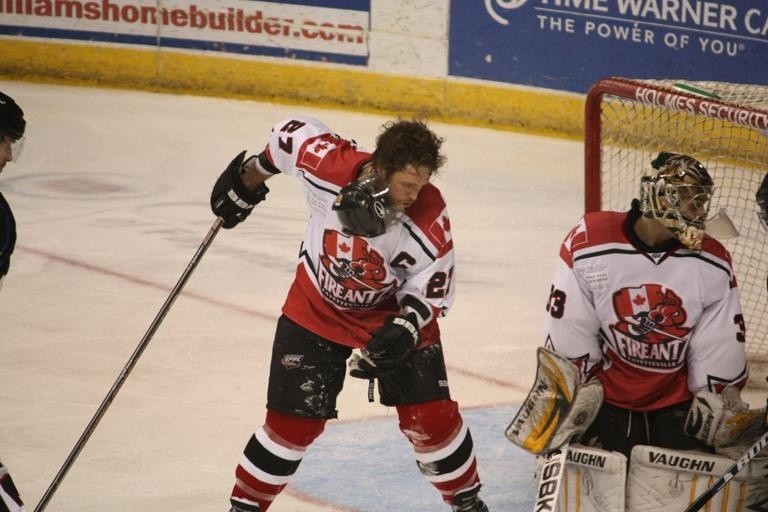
330 171 404 238
0 90 27 141
634 149 716 251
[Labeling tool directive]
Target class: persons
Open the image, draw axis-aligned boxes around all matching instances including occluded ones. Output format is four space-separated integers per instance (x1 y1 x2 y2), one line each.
0 91 27 511
504 149 767 512
211 116 490 512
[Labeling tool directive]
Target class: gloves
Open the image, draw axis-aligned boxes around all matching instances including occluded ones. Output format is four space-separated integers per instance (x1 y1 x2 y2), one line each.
207 148 272 232
344 309 422 382
